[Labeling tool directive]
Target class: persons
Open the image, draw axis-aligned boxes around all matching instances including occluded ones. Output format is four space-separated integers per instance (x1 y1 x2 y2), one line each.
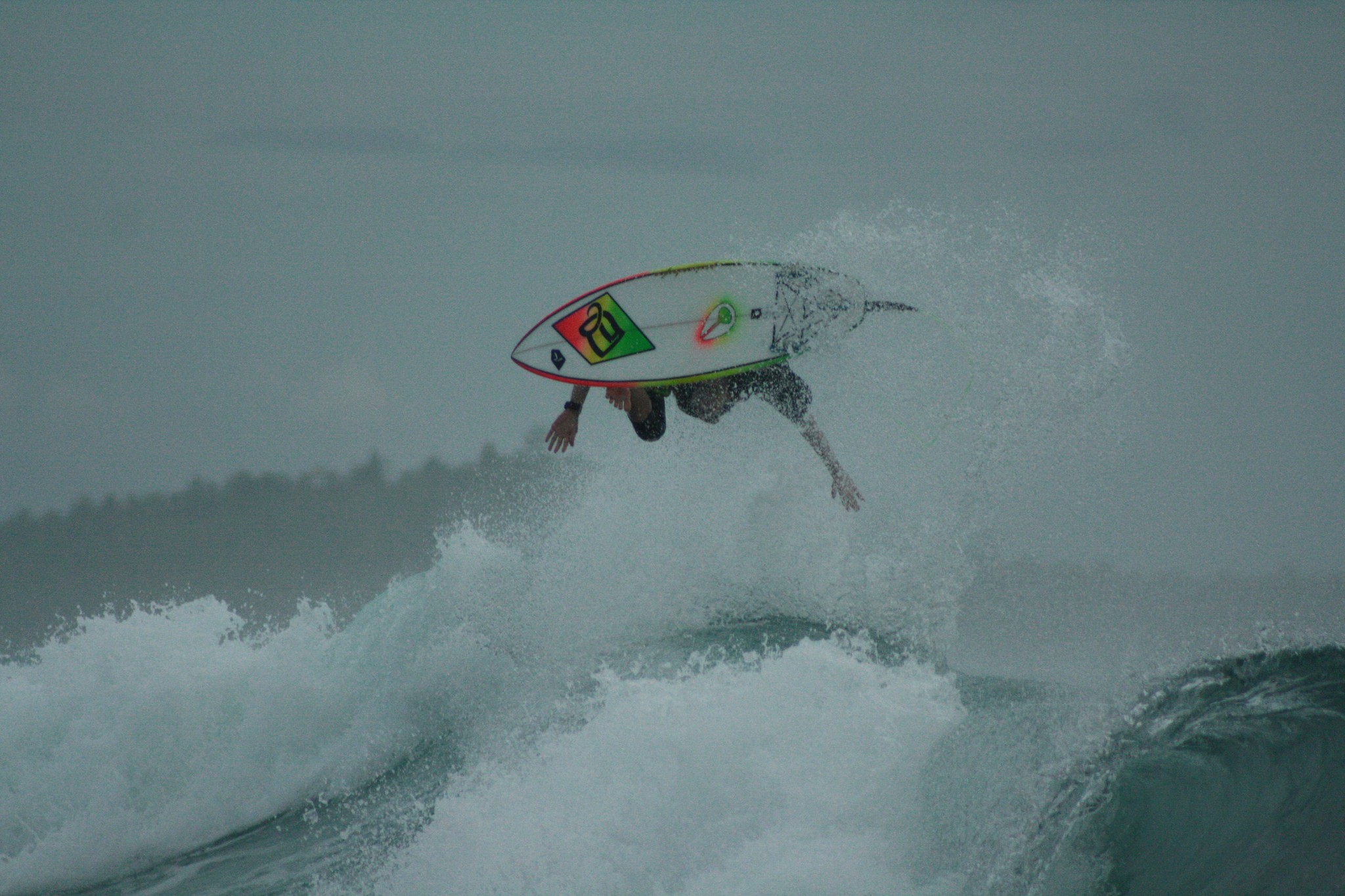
545 362 866 513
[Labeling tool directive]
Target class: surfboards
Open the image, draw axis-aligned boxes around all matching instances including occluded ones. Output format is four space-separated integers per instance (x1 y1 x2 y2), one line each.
510 260 867 388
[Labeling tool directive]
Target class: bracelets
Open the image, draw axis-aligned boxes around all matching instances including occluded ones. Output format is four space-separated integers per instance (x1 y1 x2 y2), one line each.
564 401 582 412
